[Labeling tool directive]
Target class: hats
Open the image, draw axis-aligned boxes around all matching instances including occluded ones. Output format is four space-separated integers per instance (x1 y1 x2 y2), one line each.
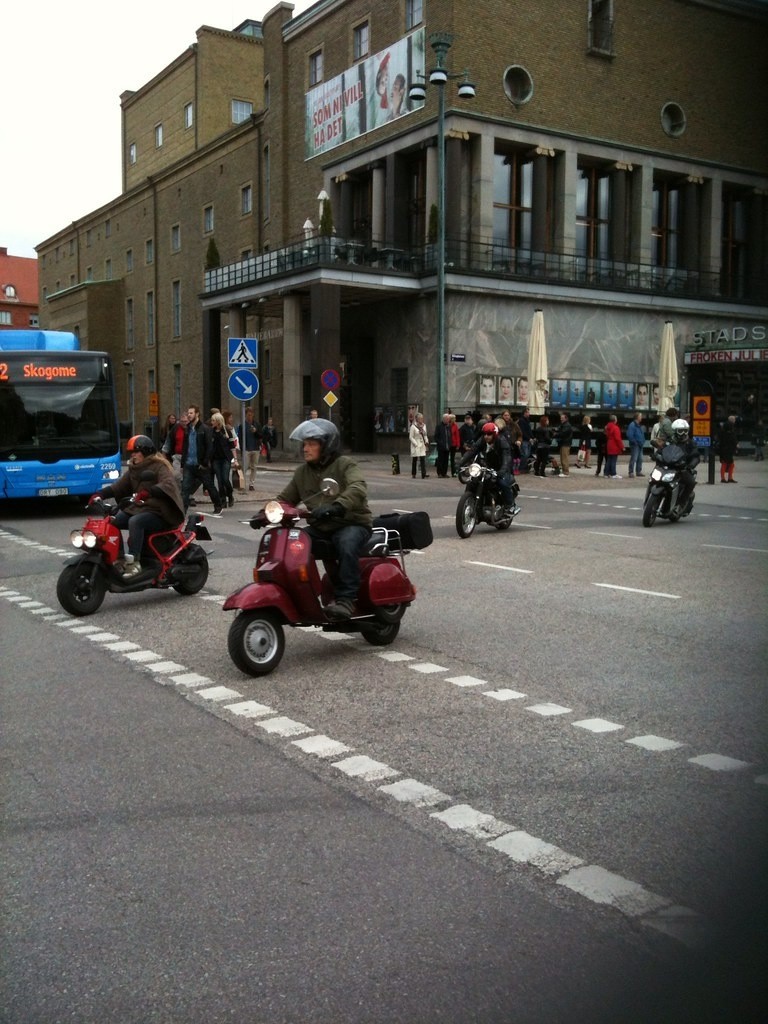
670 418 690 431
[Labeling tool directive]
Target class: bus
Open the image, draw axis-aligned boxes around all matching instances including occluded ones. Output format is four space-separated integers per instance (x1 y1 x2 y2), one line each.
0 329 123 499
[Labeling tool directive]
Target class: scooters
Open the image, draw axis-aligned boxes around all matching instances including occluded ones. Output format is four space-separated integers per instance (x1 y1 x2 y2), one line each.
57 495 214 617
223 477 418 678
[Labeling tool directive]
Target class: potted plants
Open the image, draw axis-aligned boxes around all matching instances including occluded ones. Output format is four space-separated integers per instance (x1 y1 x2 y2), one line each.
364 247 386 268
409 252 425 272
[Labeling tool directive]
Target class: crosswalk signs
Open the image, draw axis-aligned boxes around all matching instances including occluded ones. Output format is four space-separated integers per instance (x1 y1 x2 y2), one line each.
227 338 259 370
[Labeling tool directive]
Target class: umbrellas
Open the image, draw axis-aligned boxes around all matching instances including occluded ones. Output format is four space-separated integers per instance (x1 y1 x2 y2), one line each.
656 320 678 415
525 308 550 429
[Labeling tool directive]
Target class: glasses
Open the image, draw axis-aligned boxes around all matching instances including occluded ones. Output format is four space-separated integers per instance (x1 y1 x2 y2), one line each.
483 432 493 436
676 428 689 436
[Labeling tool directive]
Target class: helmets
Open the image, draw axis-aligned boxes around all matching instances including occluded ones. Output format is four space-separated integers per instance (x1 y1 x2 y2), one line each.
126 434 156 452
481 422 500 439
302 418 341 470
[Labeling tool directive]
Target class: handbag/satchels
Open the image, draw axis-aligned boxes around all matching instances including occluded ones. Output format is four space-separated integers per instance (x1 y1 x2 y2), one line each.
232 465 246 491
576 443 589 462
425 443 431 455
649 437 666 450
371 511 433 552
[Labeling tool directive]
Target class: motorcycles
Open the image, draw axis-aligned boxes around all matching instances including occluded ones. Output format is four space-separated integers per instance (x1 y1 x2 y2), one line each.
454 463 522 539
642 440 706 528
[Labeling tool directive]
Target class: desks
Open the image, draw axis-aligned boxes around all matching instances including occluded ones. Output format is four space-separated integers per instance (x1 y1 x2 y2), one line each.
339 242 365 265
380 247 405 271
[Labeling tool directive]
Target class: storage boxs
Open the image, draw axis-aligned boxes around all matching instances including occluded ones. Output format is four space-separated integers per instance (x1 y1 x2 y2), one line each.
372 511 434 550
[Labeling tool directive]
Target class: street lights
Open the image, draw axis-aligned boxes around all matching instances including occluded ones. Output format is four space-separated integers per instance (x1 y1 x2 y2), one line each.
406 31 478 468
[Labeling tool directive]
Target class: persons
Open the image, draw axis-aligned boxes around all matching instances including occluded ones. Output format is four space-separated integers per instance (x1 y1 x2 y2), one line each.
648 407 699 513
719 415 739 483
517 378 528 402
498 376 514 401
249 418 373 624
409 413 430 479
595 426 608 477
159 405 264 515
261 418 278 463
635 383 649 406
494 407 573 477
574 415 594 468
89 434 185 577
407 405 417 432
434 413 493 479
383 73 405 123
604 415 624 479
626 412 646 478
651 383 659 405
309 410 318 419
479 375 495 401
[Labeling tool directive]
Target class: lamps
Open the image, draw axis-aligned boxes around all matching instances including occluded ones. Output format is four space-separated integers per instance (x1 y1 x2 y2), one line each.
258 295 271 304
241 300 256 308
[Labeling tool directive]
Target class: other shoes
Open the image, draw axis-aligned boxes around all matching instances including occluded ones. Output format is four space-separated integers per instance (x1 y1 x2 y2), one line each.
322 596 356 621
249 486 255 491
721 479 728 483
188 497 196 507
728 479 738 483
213 496 234 516
513 467 645 479
411 473 457 479
113 554 143 580
672 504 682 517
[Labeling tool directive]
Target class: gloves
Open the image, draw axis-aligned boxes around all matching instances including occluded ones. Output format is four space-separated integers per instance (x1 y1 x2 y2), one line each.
135 489 150 504
249 509 271 529
311 503 344 523
88 493 104 506
